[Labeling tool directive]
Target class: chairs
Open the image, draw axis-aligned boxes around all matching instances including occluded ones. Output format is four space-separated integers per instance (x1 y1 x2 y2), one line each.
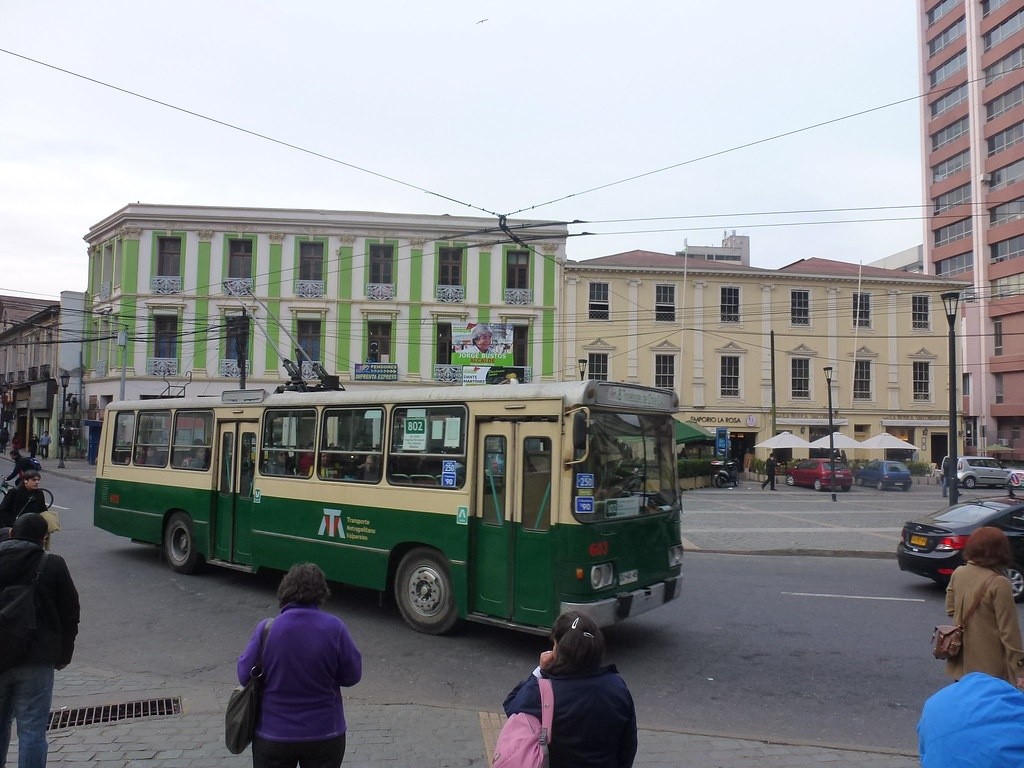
293 466 464 488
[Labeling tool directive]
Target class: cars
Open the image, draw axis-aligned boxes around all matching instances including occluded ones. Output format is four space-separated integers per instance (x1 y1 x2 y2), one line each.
941 456 1015 488
786 458 852 491
897 493 1024 604
855 460 912 491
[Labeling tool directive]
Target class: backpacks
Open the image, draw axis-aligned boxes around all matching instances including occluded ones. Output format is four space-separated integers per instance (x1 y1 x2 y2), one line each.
27 456 41 471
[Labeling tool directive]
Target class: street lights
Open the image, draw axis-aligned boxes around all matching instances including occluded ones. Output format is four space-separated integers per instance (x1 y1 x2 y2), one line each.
940 292 961 504
58 370 71 469
823 367 836 500
101 319 128 399
579 359 588 381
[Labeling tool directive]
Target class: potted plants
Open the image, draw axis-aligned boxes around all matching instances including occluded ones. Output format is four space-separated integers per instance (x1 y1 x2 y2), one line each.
747 457 938 486
677 457 719 489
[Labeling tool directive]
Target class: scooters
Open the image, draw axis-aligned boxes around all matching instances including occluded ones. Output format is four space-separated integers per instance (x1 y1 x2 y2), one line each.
711 460 740 487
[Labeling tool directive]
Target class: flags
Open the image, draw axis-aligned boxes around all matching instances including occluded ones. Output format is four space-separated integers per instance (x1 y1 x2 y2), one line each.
466 323 477 329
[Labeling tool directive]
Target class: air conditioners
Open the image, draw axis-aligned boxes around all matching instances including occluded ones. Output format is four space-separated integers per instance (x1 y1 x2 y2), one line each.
998 439 1008 447
801 425 805 434
980 173 992 181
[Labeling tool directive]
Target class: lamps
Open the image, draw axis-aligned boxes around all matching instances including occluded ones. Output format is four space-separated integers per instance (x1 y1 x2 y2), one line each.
922 427 927 435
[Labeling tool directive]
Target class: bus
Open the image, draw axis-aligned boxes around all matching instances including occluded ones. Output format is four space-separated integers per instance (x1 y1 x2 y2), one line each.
93 361 685 636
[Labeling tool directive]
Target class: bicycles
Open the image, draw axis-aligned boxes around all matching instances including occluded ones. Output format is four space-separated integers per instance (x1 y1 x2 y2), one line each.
0 476 54 510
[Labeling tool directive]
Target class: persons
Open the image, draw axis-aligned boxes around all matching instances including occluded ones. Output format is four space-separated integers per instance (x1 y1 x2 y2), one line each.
28 432 39 458
760 453 777 491
12 432 22 451
177 438 209 469
942 458 963 497
40 431 52 459
3 448 31 487
125 441 162 465
492 339 513 353
355 455 380 480
916 527 1024 768
267 445 299 475
298 442 341 479
0 512 80 768
456 326 494 353
236 562 362 768
0 426 9 452
0 470 48 527
502 611 639 768
681 448 688 459
818 447 847 466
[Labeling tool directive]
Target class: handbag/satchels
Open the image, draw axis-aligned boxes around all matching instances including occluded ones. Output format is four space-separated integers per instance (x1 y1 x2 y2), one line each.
0 526 14 542
493 679 553 768
931 625 963 659
225 620 273 754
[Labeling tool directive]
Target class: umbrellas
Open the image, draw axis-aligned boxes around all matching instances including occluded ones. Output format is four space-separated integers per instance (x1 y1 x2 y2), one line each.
673 417 715 459
752 432 920 460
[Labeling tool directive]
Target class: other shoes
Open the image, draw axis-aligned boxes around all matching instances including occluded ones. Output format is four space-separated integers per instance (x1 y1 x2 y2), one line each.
959 493 963 497
761 484 764 490
771 488 777 491
943 495 947 498
